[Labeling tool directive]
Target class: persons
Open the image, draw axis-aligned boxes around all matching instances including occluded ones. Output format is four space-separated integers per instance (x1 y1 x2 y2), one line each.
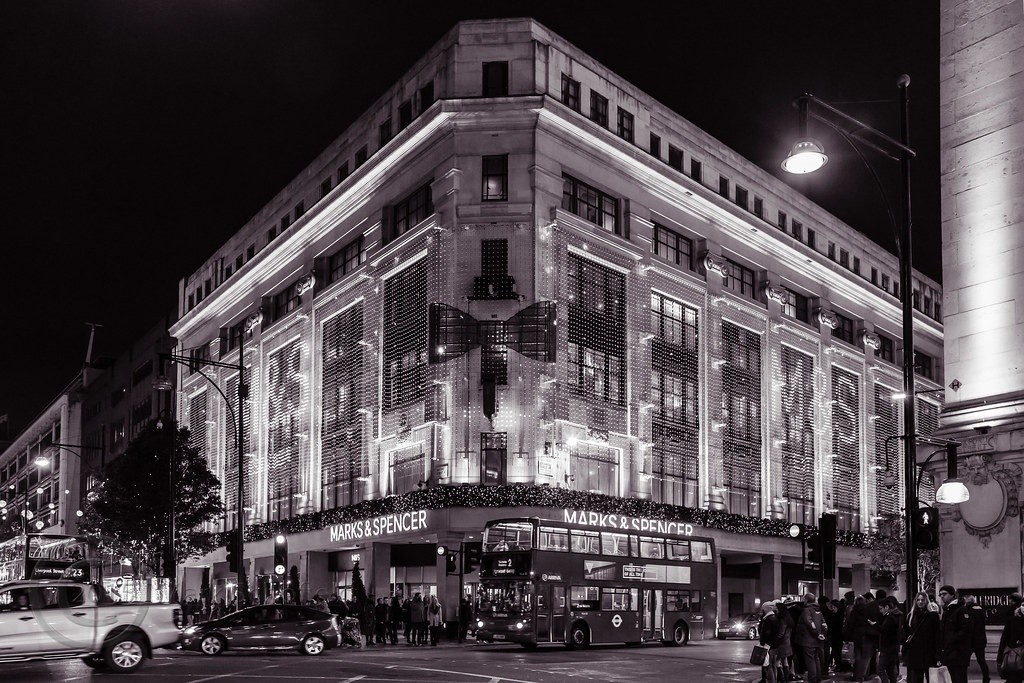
492 539 510 552
251 593 444 649
996 592 1024 683
16 594 27 606
457 598 475 643
756 587 907 683
899 582 991 683
181 596 239 626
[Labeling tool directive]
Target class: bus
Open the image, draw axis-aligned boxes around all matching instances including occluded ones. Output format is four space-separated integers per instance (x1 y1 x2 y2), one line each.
473 517 722 650
0 535 98 592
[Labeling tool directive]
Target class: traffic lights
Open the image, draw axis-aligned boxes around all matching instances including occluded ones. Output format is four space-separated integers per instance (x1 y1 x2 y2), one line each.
806 532 821 563
225 532 237 573
447 554 456 573
465 547 480 575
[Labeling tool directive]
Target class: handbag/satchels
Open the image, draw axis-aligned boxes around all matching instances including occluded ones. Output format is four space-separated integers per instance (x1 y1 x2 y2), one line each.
929 665 952 683
841 641 856 664
750 644 770 667
1000 642 1024 672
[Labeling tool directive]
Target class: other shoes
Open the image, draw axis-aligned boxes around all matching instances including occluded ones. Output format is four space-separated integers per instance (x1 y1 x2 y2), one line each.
795 675 805 683
789 675 795 683
366 638 437 646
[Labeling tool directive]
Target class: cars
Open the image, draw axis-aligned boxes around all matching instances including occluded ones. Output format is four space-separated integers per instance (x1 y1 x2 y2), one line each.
181 603 342 657
718 612 762 640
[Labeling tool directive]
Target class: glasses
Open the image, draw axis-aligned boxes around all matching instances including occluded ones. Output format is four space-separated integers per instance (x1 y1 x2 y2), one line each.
939 592 950 597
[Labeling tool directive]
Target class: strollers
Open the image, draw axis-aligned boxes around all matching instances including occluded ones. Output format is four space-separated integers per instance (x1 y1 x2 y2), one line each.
340 612 364 649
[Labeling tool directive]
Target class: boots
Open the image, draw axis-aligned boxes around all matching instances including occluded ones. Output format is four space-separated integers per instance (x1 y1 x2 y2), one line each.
777 666 784 683
784 666 789 683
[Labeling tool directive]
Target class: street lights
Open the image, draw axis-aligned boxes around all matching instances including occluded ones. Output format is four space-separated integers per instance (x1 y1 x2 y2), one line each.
33 439 105 586
789 522 806 580
275 533 288 605
438 542 463 643
151 352 246 612
0 474 28 536
778 83 971 640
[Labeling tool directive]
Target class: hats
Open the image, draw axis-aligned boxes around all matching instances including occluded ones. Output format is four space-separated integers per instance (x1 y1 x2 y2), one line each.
762 601 774 611
831 599 840 608
939 585 955 597
844 591 854 599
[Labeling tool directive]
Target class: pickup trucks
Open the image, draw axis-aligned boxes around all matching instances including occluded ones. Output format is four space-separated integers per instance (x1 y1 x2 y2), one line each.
0 579 184 675
